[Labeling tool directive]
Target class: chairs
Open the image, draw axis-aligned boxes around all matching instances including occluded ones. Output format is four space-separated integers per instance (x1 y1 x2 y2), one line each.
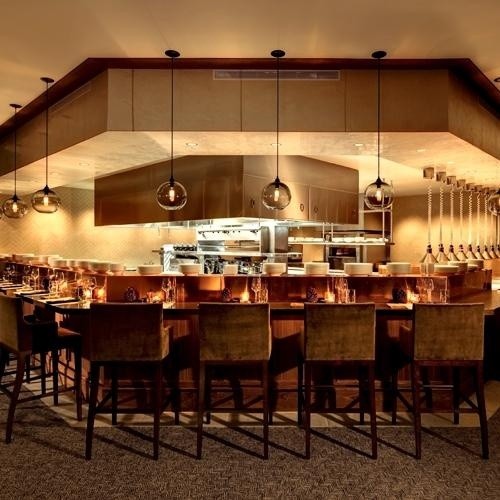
298 302 378 460
85 302 180 461
0 292 82 444
393 302 490 460
197 302 271 459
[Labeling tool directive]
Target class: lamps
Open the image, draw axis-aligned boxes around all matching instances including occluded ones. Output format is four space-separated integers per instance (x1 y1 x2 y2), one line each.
262 50 291 211
421 168 437 266
489 191 498 259
476 186 484 260
3 104 28 220
448 177 456 261
31 76 60 214
466 184 477 261
457 180 467 260
156 49 187 212
435 172 447 263
482 190 492 260
364 52 395 212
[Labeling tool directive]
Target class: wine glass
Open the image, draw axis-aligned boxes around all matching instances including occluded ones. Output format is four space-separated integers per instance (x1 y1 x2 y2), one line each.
54 271 65 295
30 267 40 290
251 277 261 302
6 263 15 283
161 276 173 303
415 278 436 303
74 271 83 301
335 278 348 304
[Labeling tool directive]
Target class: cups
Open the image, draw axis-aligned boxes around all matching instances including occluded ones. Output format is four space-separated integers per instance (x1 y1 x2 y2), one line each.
345 288 356 304
22 275 31 289
176 282 185 303
86 276 97 301
23 265 32 289
439 288 451 303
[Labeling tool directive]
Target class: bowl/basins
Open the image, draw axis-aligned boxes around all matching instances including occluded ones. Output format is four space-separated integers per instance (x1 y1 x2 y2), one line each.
89 261 111 274
27 257 40 265
386 261 412 274
66 259 77 269
343 263 374 277
468 259 484 270
448 260 468 274
12 254 34 264
434 265 460 274
137 264 164 275
467 264 479 272
53 259 66 269
263 262 287 277
223 264 240 276
110 263 126 274
0 257 6 261
304 262 330 277
180 264 200 276
78 260 91 272
5 256 13 262
47 255 56 267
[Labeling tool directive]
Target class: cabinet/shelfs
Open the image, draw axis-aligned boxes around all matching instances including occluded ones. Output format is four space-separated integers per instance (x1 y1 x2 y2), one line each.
309 185 359 224
243 174 308 221
